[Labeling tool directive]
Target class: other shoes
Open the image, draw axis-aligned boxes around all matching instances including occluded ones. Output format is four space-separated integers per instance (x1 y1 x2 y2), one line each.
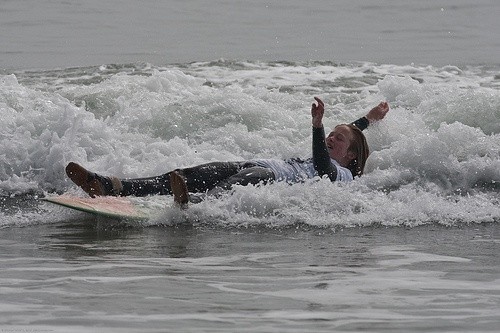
170 173 191 211
66 162 114 199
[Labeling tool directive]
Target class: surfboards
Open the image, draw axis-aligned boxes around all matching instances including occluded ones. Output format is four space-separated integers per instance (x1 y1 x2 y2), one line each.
39 197 174 220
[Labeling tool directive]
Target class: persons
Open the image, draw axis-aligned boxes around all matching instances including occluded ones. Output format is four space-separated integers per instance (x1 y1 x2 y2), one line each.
65 97 389 206
